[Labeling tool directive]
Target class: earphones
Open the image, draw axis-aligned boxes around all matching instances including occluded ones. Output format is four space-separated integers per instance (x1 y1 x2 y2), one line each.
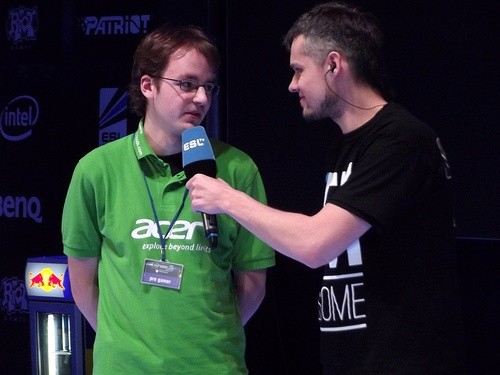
330 66 336 72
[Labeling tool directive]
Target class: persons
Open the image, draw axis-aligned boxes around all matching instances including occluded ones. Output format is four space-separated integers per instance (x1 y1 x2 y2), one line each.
186 2 464 375
60 19 277 375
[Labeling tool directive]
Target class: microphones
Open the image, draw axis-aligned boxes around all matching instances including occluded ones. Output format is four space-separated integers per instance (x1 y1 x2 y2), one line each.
182 126 218 247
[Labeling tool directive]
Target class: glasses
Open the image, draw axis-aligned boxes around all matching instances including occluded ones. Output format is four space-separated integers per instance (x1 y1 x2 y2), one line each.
155 75 219 96
